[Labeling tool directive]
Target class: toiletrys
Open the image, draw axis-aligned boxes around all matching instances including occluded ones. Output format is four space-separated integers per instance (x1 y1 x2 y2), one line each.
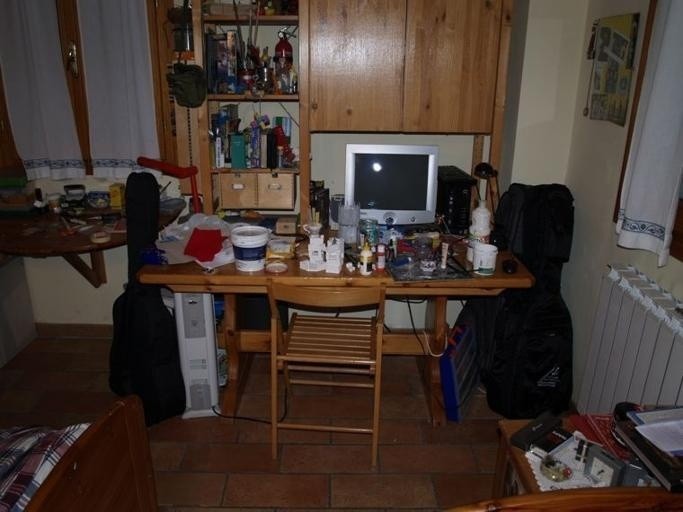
440 241 449 270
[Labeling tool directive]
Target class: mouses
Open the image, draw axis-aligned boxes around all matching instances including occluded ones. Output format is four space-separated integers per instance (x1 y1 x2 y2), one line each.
502 259 519 274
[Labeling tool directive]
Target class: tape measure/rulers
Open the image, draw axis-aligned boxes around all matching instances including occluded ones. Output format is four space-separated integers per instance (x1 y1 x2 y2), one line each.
540 459 573 483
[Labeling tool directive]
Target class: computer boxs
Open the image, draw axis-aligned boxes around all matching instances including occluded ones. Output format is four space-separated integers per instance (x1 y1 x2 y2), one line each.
435 165 472 236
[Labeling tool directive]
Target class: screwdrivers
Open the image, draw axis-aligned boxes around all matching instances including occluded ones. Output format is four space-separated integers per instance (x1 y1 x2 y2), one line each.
87 212 121 220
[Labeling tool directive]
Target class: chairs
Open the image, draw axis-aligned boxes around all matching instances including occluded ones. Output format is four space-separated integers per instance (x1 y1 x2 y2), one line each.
263 274 386 467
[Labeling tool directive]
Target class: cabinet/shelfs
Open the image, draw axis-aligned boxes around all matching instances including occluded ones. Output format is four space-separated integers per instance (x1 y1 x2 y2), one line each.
301 0 514 134
0 0 167 178
191 0 299 216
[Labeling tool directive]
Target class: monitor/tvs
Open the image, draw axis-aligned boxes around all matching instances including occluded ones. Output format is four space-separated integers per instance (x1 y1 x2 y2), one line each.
344 144 440 226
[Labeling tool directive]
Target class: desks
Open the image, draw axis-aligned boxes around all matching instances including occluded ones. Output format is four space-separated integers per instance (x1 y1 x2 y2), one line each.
135 214 536 425
0 192 186 288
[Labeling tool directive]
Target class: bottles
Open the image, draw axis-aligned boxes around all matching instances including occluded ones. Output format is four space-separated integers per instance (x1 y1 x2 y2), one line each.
472 198 490 243
358 241 386 276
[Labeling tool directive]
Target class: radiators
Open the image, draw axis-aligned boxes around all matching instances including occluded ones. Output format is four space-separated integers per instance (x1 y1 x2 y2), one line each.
575 261 683 415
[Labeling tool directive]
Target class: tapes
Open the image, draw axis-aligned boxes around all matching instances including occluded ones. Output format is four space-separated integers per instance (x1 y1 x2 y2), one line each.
90 231 112 244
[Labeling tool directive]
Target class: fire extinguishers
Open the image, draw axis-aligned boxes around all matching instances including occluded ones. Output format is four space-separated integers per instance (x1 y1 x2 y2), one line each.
274 29 297 71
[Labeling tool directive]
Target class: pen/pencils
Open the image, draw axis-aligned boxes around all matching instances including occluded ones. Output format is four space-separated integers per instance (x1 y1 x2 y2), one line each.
345 252 357 266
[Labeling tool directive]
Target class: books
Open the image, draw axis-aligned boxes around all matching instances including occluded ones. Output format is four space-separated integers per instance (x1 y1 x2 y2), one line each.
528 425 574 461
210 102 293 171
612 420 682 495
624 404 682 459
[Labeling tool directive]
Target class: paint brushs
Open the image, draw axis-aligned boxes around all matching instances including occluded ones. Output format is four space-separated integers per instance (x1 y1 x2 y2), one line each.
159 181 172 195
233 0 261 70
